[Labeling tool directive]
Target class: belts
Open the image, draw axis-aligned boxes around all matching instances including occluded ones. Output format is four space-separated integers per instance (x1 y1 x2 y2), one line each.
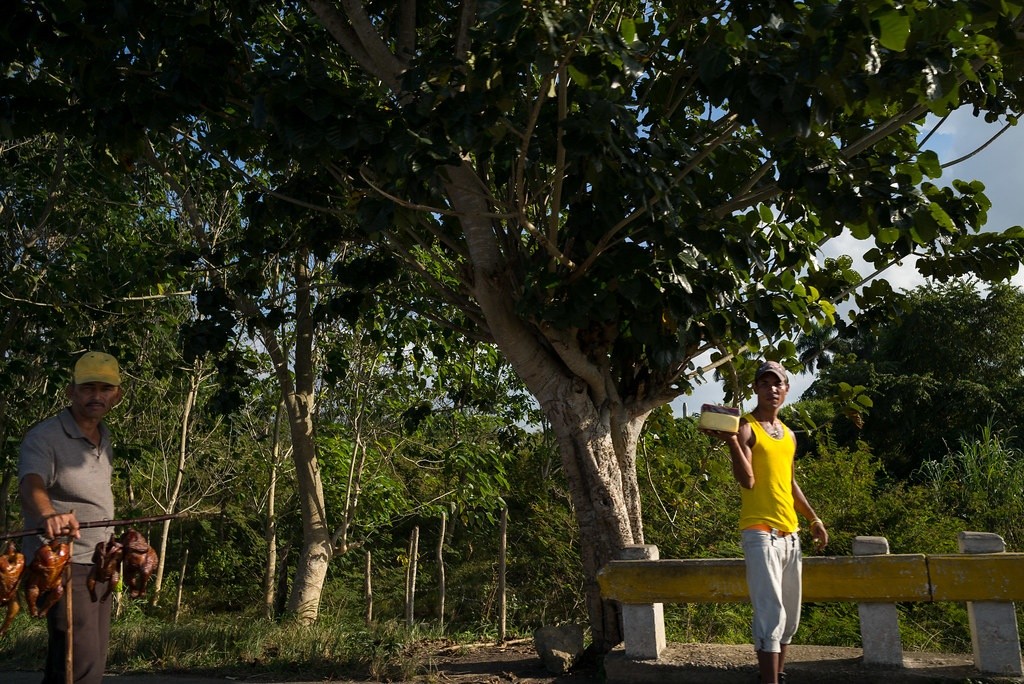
743 524 792 537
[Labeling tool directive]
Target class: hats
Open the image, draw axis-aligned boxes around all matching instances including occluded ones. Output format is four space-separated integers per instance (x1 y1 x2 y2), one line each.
755 361 787 382
73 352 122 386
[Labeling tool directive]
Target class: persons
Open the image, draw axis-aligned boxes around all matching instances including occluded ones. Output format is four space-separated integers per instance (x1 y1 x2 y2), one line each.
699 360 828 684
21 353 122 684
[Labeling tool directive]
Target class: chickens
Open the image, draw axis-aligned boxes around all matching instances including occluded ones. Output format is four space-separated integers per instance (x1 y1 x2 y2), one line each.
121 526 158 597
25 537 71 619
86 533 124 603
0 541 25 636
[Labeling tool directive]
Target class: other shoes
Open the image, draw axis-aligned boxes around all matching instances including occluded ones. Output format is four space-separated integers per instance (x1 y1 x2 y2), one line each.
778 672 787 684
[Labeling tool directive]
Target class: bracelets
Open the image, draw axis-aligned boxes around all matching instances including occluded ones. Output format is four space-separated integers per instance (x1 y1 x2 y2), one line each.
809 518 821 525
